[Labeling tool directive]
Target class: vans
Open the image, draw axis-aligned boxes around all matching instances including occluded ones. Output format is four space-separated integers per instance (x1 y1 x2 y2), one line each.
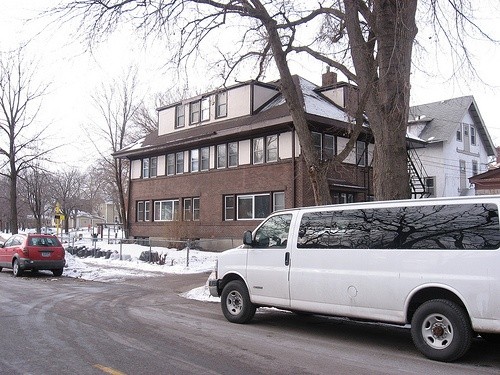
206 193 500 362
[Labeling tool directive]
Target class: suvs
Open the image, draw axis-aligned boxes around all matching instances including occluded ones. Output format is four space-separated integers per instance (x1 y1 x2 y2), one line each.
0 232 67 276
